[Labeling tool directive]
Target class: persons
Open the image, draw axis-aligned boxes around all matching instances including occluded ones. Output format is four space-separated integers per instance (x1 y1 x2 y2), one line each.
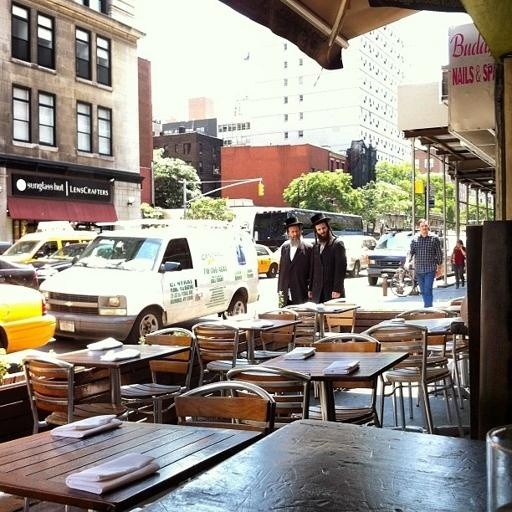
279 216 311 305
409 219 444 308
451 240 467 289
307 213 346 303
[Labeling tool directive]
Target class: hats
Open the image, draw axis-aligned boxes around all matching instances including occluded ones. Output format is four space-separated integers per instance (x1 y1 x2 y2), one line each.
308 212 333 229
283 217 305 227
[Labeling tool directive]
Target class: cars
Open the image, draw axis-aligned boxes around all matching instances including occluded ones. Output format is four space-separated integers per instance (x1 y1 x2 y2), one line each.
335 234 378 278
0 256 39 290
254 244 279 278
26 243 90 282
0 283 57 354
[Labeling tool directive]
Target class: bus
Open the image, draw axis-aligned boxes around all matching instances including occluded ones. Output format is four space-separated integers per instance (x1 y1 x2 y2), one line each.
252 208 364 253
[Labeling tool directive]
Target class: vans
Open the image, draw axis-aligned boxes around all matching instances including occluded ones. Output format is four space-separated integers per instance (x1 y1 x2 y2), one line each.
37 228 260 345
2 230 98 262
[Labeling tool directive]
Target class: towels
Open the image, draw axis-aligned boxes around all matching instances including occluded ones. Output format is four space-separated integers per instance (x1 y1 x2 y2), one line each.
50 414 123 439
86 337 123 351
251 320 274 328
323 360 361 375
64 452 159 495
283 346 317 360
99 348 141 362
391 317 405 324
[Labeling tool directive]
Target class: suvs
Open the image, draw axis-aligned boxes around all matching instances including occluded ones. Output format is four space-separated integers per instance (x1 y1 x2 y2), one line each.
366 231 417 286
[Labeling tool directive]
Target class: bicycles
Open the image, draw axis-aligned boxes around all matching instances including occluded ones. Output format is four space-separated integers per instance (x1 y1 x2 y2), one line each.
390 260 419 297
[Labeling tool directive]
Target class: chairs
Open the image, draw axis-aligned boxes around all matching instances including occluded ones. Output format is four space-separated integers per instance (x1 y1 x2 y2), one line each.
121 327 195 426
394 309 454 422
241 308 298 394
175 381 274 434
22 355 126 512
292 309 318 398
324 298 358 341
367 323 467 438
425 296 469 396
227 365 310 431
194 321 262 423
306 335 381 428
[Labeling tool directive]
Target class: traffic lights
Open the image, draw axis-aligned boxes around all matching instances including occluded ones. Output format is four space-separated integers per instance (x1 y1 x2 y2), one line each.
258 184 265 196
